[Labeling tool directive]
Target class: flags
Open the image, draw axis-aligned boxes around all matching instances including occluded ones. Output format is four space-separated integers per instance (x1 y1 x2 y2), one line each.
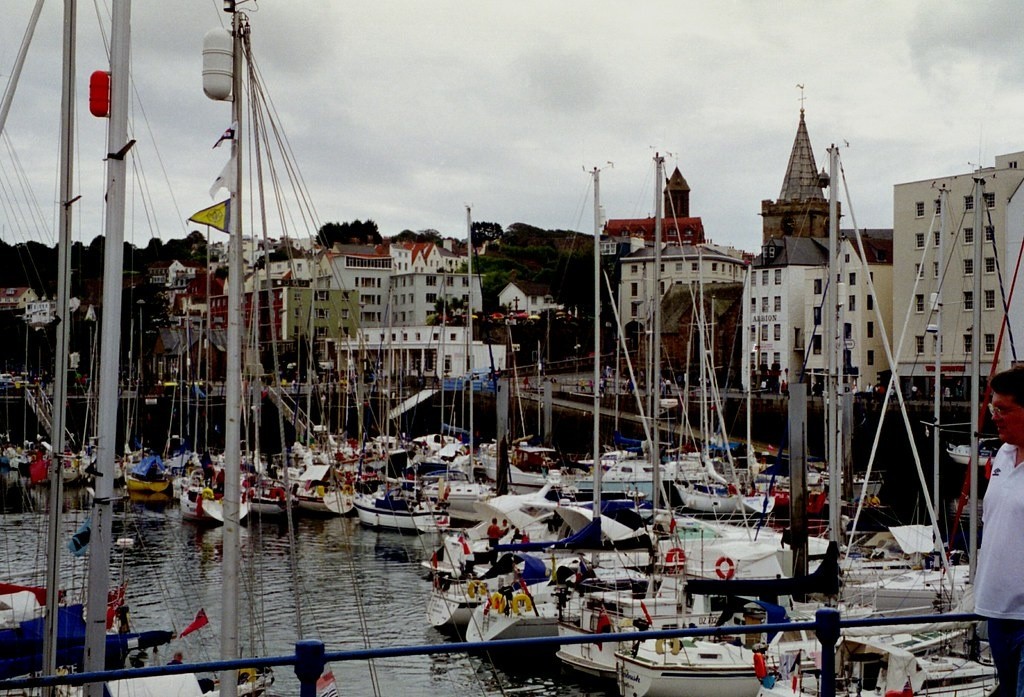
316 671 339 697
457 534 470 555
66 516 92 556
593 605 612 652
669 517 677 535
180 608 209 638
565 560 589 584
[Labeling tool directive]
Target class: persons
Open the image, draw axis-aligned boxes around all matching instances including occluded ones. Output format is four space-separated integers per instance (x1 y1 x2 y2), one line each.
520 366 684 399
740 482 748 496
167 652 183 665
972 366 1024 697
653 521 665 532
216 469 225 493
115 600 129 634
487 518 531 547
760 375 963 401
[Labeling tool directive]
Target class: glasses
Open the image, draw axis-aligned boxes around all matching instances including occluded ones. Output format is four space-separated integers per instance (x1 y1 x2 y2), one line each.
988 403 1022 415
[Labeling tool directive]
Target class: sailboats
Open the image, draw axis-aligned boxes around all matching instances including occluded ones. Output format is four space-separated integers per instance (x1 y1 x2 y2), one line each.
0 0 278 697
203 165 748 673
612 145 1024 696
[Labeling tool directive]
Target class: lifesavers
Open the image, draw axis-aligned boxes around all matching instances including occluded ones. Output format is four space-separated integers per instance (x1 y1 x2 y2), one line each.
202 488 215 500
491 592 505 615
752 652 768 677
654 638 680 656
512 592 532 615
665 547 686 571
715 556 734 579
467 580 486 599
